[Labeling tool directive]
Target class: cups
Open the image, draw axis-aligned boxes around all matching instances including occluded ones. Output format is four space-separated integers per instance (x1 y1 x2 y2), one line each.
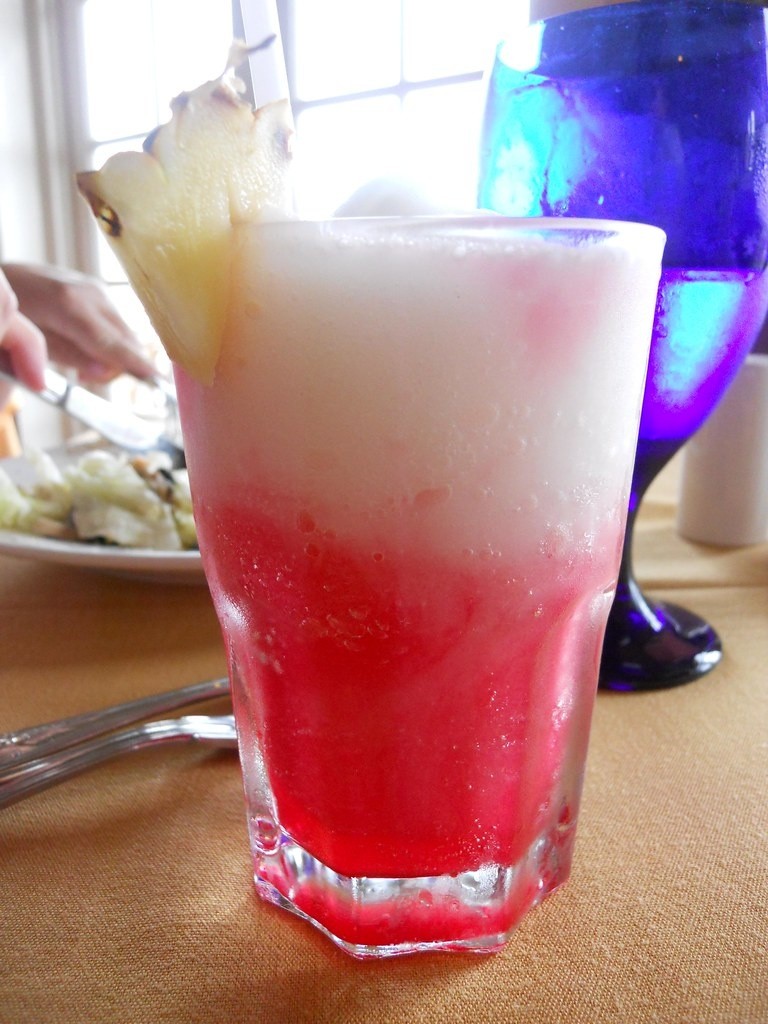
165 214 667 959
677 354 768 547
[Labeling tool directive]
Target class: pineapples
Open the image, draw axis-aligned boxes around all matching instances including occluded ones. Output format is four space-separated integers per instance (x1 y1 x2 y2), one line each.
76 77 295 389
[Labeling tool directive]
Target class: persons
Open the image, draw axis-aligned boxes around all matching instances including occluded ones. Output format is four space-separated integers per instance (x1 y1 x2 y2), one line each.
1 259 151 391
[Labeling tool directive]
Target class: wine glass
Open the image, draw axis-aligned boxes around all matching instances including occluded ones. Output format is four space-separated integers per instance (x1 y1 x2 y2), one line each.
472 0 768 693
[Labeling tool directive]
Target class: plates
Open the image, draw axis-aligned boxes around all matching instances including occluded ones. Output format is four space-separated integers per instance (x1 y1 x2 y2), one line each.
0 435 210 589
